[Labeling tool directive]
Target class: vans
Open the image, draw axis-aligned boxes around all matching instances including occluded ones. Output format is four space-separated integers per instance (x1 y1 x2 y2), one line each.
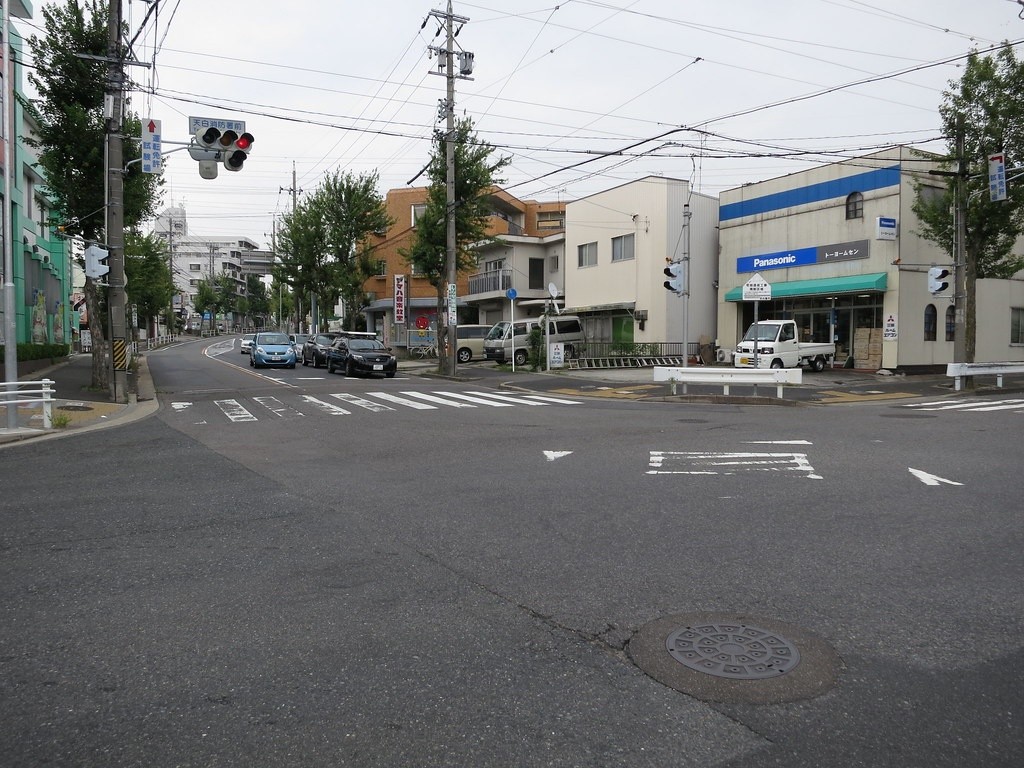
444 324 510 363
482 315 588 365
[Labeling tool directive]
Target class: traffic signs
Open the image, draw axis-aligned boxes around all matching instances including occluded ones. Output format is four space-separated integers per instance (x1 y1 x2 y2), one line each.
988 152 1007 202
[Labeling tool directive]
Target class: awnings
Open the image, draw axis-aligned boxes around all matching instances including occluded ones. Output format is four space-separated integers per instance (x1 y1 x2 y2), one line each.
724 272 888 302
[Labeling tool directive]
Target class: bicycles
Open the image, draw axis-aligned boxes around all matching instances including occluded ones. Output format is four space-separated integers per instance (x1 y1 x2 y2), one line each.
411 338 437 360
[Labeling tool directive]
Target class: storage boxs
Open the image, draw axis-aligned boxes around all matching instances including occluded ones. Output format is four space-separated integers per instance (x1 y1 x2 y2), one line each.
854 328 883 369
836 345 848 361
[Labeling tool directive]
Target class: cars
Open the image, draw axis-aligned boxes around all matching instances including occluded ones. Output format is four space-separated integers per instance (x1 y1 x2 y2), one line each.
290 331 397 378
239 332 296 368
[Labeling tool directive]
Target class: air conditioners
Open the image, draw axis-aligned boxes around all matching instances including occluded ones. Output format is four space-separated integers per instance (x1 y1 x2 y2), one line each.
716 348 734 363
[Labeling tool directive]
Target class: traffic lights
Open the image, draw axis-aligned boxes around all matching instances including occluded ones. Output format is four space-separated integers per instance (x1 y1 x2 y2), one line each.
929 268 950 294
196 127 255 172
663 263 683 293
85 246 111 279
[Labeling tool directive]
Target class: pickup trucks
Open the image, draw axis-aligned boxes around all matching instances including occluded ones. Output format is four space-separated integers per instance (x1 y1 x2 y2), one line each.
733 319 836 371
